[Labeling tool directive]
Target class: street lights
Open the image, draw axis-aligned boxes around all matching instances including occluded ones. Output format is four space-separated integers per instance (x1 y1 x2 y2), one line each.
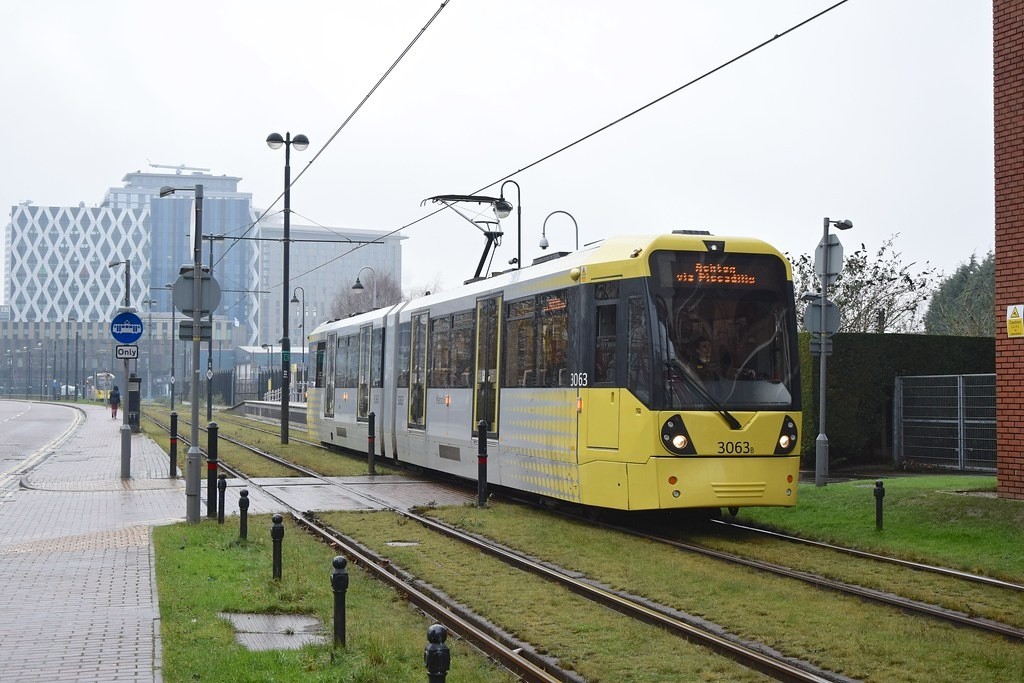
165 280 182 415
498 182 524 264
37 341 46 402
541 209 581 258
266 130 310 449
201 232 225 421
142 299 156 405
815 215 854 487
353 266 378 311
107 257 131 432
292 286 308 404
7 348 11 397
64 317 71 401
261 343 273 401
23 346 30 400
158 185 203 526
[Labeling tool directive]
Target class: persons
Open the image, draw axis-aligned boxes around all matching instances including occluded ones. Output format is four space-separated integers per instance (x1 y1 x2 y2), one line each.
689 338 755 382
109 386 121 419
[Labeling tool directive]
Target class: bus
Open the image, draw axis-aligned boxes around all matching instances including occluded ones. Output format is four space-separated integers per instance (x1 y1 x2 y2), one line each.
86 371 117 401
304 222 802 516
